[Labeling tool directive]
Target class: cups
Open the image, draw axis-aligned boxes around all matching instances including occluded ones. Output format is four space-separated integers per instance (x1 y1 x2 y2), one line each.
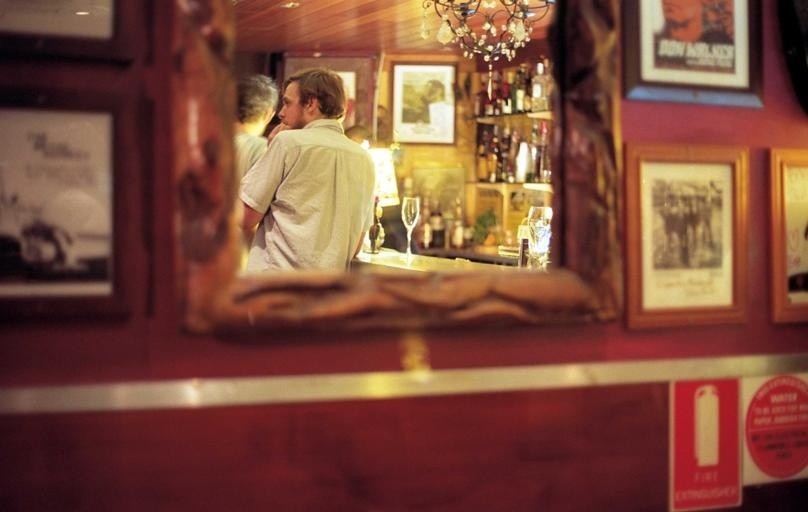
525 204 552 269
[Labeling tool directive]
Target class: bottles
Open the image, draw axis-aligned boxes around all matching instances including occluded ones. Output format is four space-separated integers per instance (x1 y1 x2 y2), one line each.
473 55 550 182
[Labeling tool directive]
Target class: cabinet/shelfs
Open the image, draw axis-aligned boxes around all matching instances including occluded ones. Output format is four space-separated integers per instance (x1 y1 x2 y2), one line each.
474 36 552 253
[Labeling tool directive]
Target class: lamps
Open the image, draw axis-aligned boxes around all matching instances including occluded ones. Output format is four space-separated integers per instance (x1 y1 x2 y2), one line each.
419 0 558 101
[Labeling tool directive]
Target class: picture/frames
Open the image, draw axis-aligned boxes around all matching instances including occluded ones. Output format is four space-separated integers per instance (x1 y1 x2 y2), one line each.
1 0 140 66
621 0 765 108
0 82 145 321
769 148 808 324
390 61 459 145
625 143 750 329
279 53 376 138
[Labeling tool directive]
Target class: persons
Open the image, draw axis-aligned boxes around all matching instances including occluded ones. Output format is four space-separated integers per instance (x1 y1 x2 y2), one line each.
238 69 376 267
232 61 280 262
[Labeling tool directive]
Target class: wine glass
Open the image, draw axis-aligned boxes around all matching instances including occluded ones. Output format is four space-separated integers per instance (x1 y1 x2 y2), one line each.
400 194 422 259
